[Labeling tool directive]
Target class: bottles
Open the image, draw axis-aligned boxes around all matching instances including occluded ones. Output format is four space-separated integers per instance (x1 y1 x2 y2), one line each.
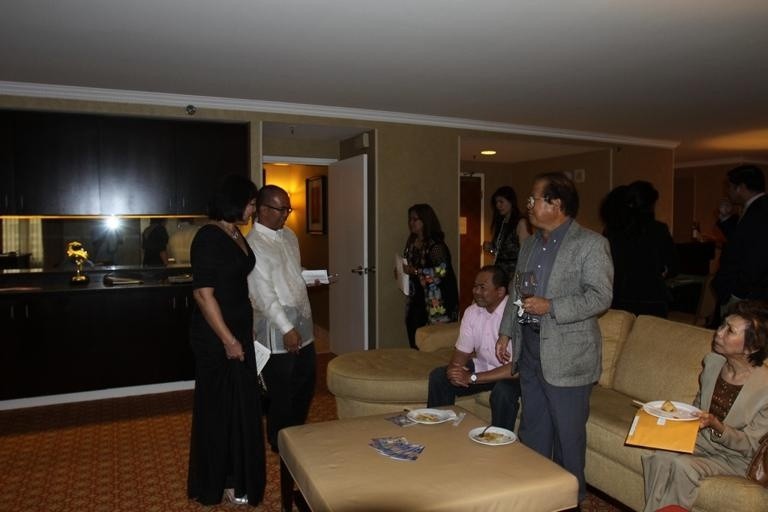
691 219 701 240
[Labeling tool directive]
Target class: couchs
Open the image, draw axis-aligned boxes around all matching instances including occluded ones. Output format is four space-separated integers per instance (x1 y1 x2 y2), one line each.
325 298 768 511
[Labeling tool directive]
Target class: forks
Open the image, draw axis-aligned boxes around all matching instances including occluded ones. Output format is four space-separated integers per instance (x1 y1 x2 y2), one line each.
475 423 494 437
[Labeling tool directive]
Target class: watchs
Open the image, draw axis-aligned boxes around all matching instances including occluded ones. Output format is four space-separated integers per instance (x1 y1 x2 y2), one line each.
470 372 478 384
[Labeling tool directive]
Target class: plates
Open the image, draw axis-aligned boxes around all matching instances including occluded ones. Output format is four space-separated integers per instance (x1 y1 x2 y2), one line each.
641 401 703 421
406 407 457 426
469 425 517 446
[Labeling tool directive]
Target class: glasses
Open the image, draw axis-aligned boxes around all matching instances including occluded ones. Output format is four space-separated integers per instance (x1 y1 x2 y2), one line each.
528 195 542 208
267 205 292 214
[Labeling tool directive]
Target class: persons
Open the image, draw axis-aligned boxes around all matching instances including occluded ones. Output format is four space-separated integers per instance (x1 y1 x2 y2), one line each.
249 185 326 457
425 264 520 432
138 218 175 269
636 299 767 511
188 179 274 509
392 202 460 352
592 164 768 327
493 171 619 512
482 189 534 295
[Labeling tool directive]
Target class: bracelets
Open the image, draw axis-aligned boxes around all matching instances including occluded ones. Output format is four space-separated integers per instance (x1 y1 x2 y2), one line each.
414 266 418 275
222 336 239 347
710 425 726 437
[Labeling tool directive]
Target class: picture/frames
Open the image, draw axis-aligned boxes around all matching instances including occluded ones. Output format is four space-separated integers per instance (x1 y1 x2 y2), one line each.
304 175 328 237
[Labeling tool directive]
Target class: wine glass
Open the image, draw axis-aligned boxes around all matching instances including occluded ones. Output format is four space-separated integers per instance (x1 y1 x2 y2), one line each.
516 271 540 324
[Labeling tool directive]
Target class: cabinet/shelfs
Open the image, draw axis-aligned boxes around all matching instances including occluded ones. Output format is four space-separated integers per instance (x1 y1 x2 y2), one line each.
0 107 101 218
101 113 252 219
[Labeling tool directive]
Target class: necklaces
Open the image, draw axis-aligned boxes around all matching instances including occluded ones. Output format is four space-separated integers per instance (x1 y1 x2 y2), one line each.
219 221 239 239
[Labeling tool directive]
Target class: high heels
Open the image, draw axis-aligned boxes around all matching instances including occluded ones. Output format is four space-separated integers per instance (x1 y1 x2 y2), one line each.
221 487 248 504
